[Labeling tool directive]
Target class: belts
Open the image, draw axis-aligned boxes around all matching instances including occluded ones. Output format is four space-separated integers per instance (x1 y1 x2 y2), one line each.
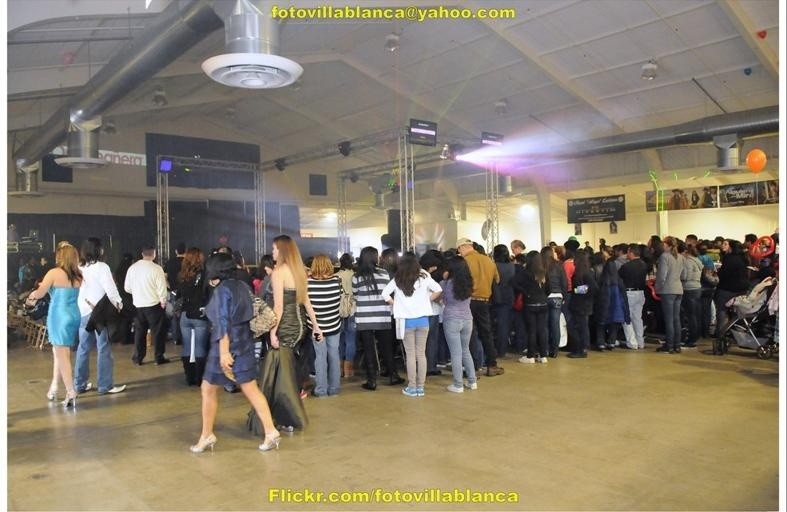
625 288 645 292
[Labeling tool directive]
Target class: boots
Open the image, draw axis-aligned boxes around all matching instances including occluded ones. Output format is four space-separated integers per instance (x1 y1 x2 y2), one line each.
344 360 354 378
340 360 344 377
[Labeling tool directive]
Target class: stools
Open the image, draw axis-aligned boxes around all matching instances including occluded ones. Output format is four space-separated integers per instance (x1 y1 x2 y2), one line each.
24 318 49 350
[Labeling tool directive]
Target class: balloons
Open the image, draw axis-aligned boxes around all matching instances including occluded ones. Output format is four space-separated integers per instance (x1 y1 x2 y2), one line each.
744 148 766 174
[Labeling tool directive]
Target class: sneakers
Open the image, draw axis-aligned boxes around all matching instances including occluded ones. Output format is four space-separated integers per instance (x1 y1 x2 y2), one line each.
402 386 417 397
656 346 674 354
464 383 478 390
674 347 682 354
518 355 536 365
277 424 295 432
299 389 308 400
427 370 442 376
536 355 548 363
567 351 588 358
361 377 376 390
416 386 424 397
391 376 406 386
487 365 505 377
446 384 464 394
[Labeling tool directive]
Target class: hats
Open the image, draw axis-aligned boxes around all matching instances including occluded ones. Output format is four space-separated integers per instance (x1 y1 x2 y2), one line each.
456 238 473 255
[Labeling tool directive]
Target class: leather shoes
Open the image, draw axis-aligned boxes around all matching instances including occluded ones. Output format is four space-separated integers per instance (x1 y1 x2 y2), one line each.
107 385 127 394
84 382 93 391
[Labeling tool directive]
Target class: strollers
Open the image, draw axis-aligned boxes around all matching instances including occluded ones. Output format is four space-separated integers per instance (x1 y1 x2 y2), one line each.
713 276 777 358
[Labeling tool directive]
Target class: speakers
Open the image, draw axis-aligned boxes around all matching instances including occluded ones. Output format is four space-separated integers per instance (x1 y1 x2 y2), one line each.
41 154 76 183
387 208 405 241
308 173 329 195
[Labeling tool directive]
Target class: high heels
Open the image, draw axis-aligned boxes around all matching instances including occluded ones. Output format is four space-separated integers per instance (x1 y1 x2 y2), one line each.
47 382 57 401
259 430 280 451
190 434 218 454
61 387 77 407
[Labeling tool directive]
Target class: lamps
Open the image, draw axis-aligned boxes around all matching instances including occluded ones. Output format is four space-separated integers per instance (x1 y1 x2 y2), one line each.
383 28 402 56
349 172 358 182
641 58 659 80
103 119 116 135
158 158 172 172
150 84 167 106
274 159 285 172
439 144 457 161
493 98 508 114
337 141 351 156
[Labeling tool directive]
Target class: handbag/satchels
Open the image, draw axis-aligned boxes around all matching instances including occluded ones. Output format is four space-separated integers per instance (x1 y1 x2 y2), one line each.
249 297 278 341
339 293 353 319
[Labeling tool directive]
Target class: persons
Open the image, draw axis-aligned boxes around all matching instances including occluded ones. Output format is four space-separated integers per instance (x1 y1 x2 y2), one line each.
6 219 779 455
701 187 709 209
705 186 717 208
668 189 689 209
692 191 700 209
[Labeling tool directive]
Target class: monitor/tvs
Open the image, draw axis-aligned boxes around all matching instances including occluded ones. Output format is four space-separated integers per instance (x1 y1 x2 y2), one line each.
480 130 506 156
408 117 438 149
